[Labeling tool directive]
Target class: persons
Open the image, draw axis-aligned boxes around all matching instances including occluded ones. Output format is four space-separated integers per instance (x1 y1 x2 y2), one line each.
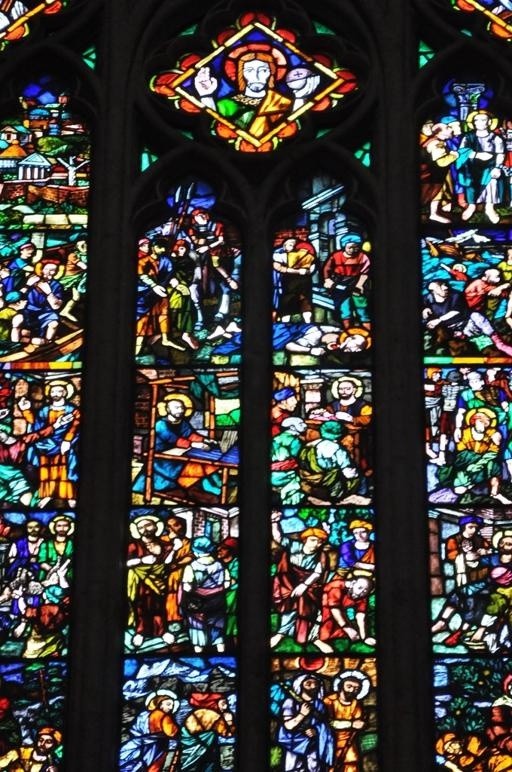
194 44 320 141
